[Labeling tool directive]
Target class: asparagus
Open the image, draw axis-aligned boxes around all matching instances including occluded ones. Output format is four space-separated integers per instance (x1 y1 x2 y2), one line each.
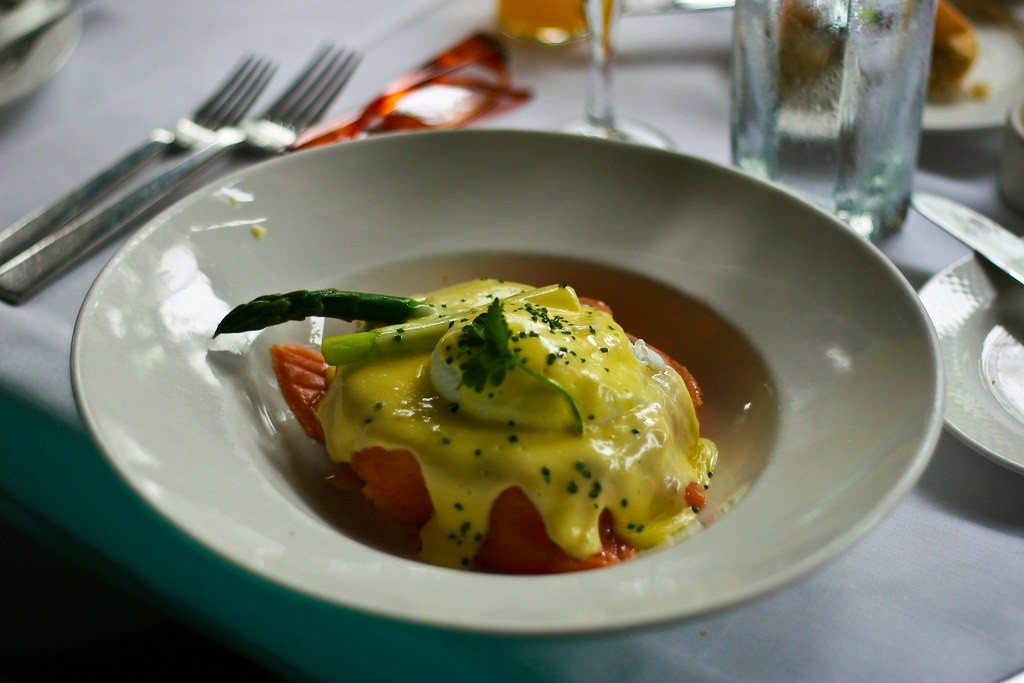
213 289 436 338
322 283 580 366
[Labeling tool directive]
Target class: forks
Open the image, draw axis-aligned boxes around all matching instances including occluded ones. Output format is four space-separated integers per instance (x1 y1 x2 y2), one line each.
0 42 362 306
1 55 278 264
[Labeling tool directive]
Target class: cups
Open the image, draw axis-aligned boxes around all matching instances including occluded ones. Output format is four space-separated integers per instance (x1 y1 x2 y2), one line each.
733 0 938 244
1001 96 1024 209
497 0 592 45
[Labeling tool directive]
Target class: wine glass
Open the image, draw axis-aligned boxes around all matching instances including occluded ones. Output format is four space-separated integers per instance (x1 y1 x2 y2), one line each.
560 0 674 152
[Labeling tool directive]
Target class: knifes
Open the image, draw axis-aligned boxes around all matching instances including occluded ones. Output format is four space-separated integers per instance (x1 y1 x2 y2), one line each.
911 190 1024 283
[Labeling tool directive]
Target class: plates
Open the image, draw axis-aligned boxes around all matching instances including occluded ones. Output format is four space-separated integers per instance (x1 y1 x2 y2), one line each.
70 133 943 635
925 22 1024 130
918 251 1024 476
0 0 83 104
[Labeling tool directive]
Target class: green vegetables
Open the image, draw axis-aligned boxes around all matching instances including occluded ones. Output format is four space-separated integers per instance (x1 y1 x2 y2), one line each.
455 297 584 434
